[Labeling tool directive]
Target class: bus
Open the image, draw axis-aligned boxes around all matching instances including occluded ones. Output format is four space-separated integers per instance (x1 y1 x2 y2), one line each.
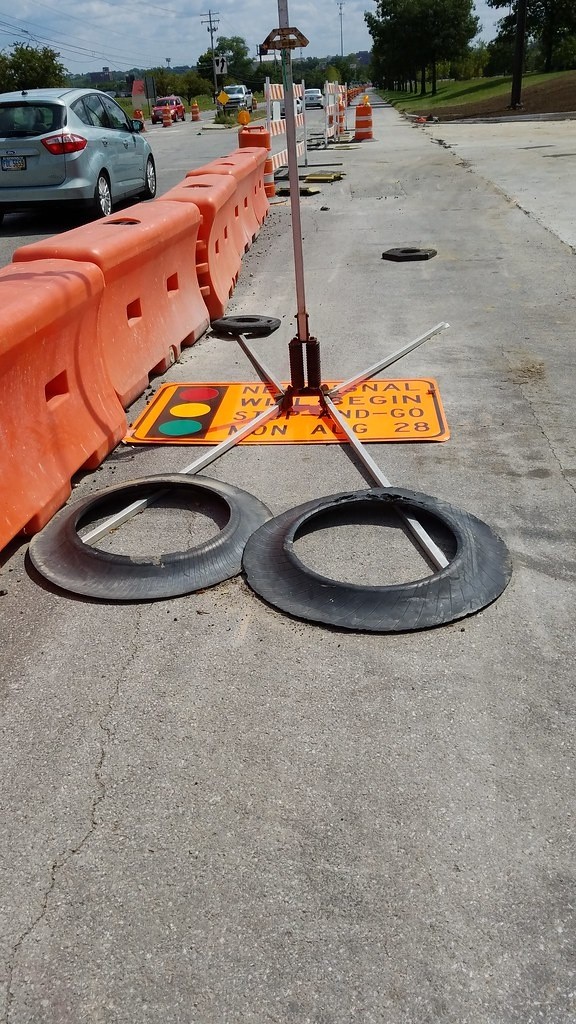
218 84 255 114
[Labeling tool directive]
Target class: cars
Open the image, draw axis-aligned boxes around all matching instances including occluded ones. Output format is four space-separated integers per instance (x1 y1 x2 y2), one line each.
1 88 158 224
151 96 186 123
274 96 304 117
305 89 325 109
